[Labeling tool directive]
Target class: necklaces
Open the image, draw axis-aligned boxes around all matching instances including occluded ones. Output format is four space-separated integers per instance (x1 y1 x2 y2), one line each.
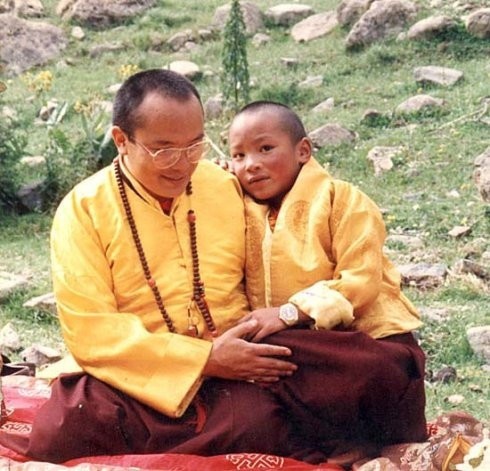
113 157 218 433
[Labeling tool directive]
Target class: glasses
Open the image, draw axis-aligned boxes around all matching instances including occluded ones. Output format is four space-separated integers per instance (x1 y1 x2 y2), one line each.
128 134 214 170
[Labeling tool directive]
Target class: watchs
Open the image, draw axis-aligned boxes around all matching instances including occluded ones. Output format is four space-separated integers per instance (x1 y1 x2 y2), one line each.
278 304 299 328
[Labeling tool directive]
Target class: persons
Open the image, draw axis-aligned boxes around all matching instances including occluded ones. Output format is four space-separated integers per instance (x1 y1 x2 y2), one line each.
205 101 427 459
27 69 291 462
